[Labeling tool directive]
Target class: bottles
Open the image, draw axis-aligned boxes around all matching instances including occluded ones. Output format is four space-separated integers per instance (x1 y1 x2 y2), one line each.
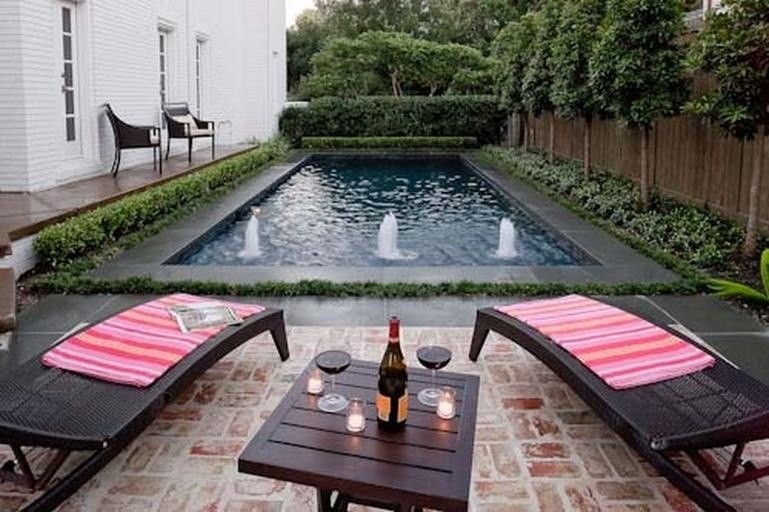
374 316 409 435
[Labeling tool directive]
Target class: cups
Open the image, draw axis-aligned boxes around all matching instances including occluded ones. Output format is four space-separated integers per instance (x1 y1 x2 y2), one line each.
305 367 324 396
435 385 458 422
344 398 368 432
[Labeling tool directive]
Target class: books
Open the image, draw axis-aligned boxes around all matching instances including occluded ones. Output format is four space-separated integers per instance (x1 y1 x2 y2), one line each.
164 301 244 336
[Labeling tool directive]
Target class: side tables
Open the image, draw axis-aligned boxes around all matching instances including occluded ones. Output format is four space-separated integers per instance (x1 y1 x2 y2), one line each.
237 354 480 511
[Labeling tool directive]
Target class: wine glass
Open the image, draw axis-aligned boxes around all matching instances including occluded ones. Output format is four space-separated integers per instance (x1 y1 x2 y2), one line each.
311 328 353 414
415 328 453 405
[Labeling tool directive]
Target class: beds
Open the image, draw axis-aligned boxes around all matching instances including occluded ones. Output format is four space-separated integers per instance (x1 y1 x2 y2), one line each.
471 289 769 511
1 292 291 511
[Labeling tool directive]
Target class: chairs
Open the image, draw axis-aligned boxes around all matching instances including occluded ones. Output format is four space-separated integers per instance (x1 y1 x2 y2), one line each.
104 103 163 177
162 100 215 163
